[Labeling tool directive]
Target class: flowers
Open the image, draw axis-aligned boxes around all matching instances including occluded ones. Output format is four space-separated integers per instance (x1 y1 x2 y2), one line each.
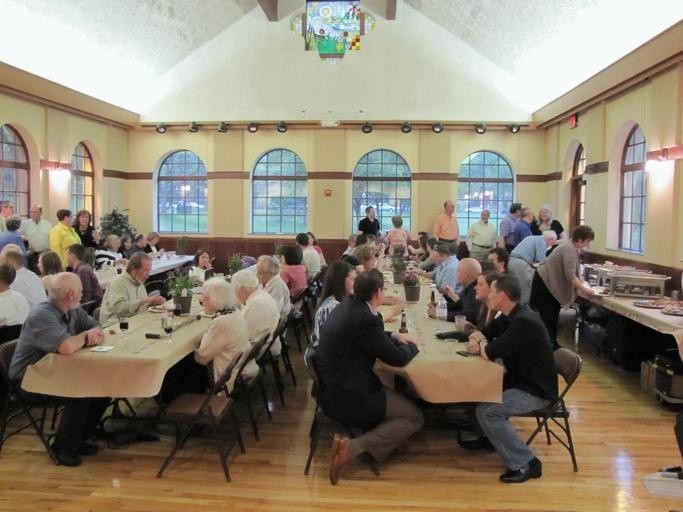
398 265 426 285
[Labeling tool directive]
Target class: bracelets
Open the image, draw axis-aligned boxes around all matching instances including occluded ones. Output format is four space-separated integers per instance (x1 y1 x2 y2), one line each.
478 339 488 344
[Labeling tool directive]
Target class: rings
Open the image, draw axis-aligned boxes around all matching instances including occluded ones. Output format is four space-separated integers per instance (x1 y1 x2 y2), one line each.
159 299 162 302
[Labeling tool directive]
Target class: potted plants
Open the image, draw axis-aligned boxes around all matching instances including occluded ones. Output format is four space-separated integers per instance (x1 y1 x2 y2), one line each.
164 266 197 313
392 242 406 257
389 257 407 284
226 249 243 276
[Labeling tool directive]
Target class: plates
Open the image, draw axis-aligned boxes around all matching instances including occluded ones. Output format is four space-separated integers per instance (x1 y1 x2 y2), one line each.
593 286 616 297
634 301 662 309
663 307 683 316
148 306 164 313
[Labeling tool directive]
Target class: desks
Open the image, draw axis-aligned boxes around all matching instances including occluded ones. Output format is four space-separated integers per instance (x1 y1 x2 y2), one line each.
578 282 683 403
21 282 215 446
375 269 505 446
96 248 195 290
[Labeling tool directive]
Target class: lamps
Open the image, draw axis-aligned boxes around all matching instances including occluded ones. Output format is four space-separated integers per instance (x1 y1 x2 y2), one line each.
288 0 377 69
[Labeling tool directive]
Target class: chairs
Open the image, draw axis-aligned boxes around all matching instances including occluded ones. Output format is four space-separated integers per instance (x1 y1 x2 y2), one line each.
505 346 583 473
304 348 380 476
0 324 22 449
311 329 318 350
557 303 579 353
80 300 95 316
156 351 246 482
1 338 63 466
144 279 170 298
231 264 327 440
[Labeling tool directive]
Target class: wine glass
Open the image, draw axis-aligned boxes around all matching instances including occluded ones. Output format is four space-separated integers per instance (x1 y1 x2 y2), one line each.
163 318 173 344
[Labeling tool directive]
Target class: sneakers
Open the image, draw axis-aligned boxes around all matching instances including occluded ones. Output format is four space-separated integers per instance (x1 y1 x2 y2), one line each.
49 443 80 465
79 442 103 454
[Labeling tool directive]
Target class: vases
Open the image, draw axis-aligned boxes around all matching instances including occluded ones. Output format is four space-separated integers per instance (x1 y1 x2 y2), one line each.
404 286 420 301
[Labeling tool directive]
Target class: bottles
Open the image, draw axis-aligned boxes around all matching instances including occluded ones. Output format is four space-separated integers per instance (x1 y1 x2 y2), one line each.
399 313 408 334
671 290 680 300
429 290 436 318
160 308 168 334
435 296 448 332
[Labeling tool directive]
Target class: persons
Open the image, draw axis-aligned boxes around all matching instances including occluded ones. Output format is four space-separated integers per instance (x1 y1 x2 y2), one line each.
467 275 562 485
155 278 260 436
9 271 104 466
255 255 291 326
315 258 357 344
189 248 215 283
329 200 611 320
295 232 321 282
314 268 424 485
232 269 283 357
306 232 327 266
0 200 165 341
277 244 308 311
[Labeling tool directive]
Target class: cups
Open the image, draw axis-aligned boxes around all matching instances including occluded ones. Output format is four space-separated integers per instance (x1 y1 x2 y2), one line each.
174 304 182 318
445 338 458 358
119 316 129 332
454 315 466 332
166 304 176 319
116 265 123 275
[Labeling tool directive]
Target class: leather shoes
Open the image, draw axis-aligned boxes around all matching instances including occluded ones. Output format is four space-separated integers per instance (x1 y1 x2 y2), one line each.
459 435 494 454
330 430 351 485
499 455 547 482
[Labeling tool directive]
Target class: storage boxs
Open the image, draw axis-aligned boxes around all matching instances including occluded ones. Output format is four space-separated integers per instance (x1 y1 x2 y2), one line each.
641 362 682 398
